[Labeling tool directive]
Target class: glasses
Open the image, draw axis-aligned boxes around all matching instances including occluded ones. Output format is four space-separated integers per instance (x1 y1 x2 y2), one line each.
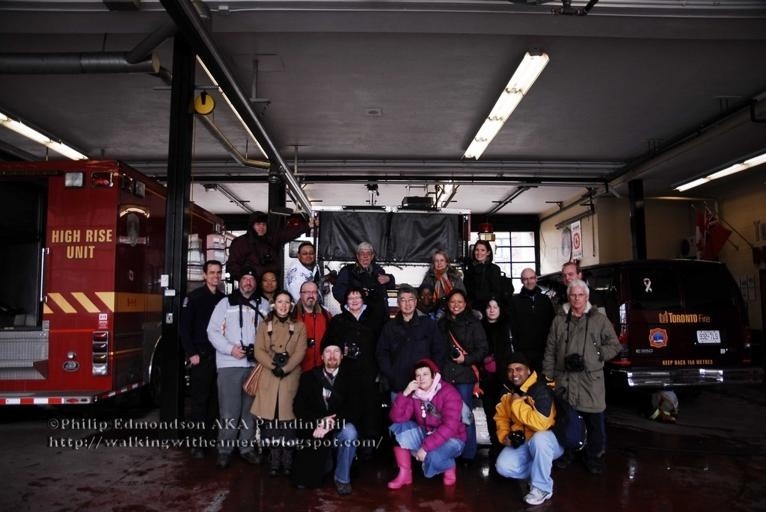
240 276 254 282
300 290 318 296
522 276 537 281
399 297 416 303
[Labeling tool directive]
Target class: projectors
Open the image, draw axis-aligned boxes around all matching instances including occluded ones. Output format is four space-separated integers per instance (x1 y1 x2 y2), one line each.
402 196 433 208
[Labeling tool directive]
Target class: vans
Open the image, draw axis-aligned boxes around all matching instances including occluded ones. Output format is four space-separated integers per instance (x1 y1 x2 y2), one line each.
519 257 754 393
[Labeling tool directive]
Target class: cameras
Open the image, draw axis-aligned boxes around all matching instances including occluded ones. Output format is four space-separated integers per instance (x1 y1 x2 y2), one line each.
508 429 525 449
307 338 315 347
567 353 581 370
242 344 255 362
347 343 361 360
451 347 459 358
273 351 288 366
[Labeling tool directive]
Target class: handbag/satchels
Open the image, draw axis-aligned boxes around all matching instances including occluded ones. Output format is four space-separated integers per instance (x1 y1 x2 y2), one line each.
460 402 474 426
241 364 263 395
471 365 484 398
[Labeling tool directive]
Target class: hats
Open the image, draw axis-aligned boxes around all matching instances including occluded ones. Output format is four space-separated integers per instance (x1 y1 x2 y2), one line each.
506 349 526 364
320 331 345 353
240 268 257 276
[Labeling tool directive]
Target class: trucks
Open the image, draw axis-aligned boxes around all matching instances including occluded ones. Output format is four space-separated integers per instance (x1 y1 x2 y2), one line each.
282 234 313 301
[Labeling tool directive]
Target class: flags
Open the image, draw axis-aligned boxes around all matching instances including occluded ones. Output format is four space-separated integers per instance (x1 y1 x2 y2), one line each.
691 208 732 261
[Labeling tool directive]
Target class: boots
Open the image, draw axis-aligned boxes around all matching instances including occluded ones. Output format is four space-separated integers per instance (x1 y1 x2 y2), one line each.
388 447 412 489
444 464 457 484
267 445 279 476
283 444 292 478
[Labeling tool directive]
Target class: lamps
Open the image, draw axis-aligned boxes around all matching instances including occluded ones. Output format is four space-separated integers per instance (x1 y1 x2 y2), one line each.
671 149 766 193
0 106 93 161
462 44 552 161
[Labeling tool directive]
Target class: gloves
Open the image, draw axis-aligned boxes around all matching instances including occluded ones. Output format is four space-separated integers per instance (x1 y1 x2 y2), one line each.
272 364 285 376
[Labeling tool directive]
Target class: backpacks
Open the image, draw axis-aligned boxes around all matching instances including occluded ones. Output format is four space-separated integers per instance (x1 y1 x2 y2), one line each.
526 384 588 454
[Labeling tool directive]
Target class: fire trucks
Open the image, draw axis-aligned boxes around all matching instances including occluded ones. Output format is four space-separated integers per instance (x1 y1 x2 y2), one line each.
0 159 241 407
312 195 496 325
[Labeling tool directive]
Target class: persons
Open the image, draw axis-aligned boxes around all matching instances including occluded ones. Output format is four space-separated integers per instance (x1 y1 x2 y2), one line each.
228 212 320 293
177 239 623 506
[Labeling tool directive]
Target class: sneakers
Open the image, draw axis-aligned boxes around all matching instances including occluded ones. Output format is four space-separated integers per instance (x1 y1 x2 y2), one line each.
335 479 351 494
242 450 264 465
216 453 230 466
297 472 324 489
523 486 553 506
